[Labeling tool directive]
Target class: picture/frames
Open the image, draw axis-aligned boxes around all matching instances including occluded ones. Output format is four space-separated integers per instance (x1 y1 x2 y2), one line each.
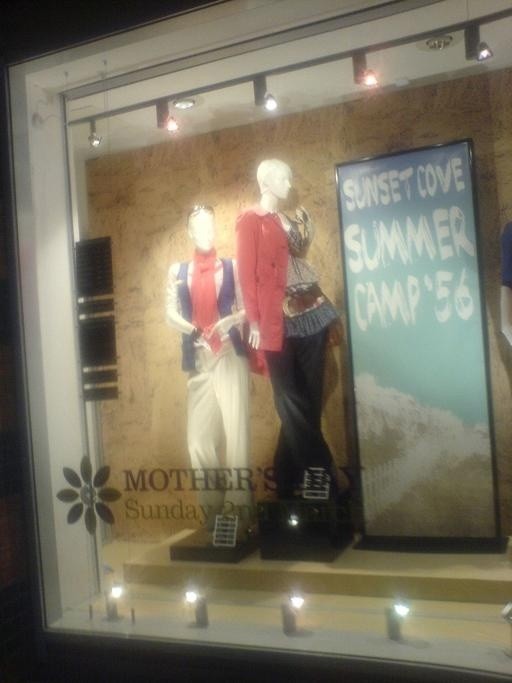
335 137 509 554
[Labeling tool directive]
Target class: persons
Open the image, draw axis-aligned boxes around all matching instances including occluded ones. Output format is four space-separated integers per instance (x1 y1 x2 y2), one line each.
160 204 252 535
498 221 511 348
236 158 352 504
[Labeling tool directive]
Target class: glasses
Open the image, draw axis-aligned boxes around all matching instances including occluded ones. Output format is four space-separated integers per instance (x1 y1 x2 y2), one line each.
189 204 216 214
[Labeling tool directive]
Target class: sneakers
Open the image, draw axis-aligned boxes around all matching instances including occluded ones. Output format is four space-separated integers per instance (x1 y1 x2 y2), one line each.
342 464 361 501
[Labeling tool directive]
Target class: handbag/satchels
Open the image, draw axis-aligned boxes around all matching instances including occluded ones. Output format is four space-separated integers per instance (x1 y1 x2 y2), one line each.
284 282 337 338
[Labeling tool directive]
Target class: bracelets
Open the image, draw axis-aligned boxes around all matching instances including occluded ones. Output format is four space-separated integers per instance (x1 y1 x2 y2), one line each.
189 327 202 342
